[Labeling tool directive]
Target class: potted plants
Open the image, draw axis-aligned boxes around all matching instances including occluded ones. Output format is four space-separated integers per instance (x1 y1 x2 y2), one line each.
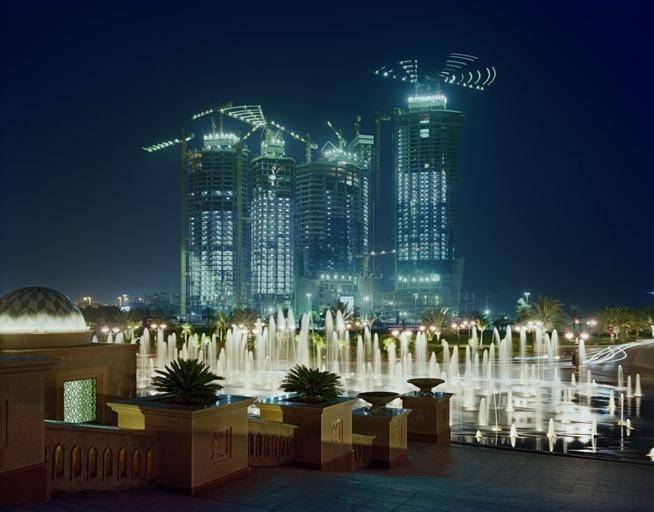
253 363 359 471
106 357 258 495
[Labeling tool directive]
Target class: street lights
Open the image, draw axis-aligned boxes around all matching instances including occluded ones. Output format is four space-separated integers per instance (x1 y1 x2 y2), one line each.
83 296 91 305
524 292 531 303
306 293 311 309
118 297 121 306
